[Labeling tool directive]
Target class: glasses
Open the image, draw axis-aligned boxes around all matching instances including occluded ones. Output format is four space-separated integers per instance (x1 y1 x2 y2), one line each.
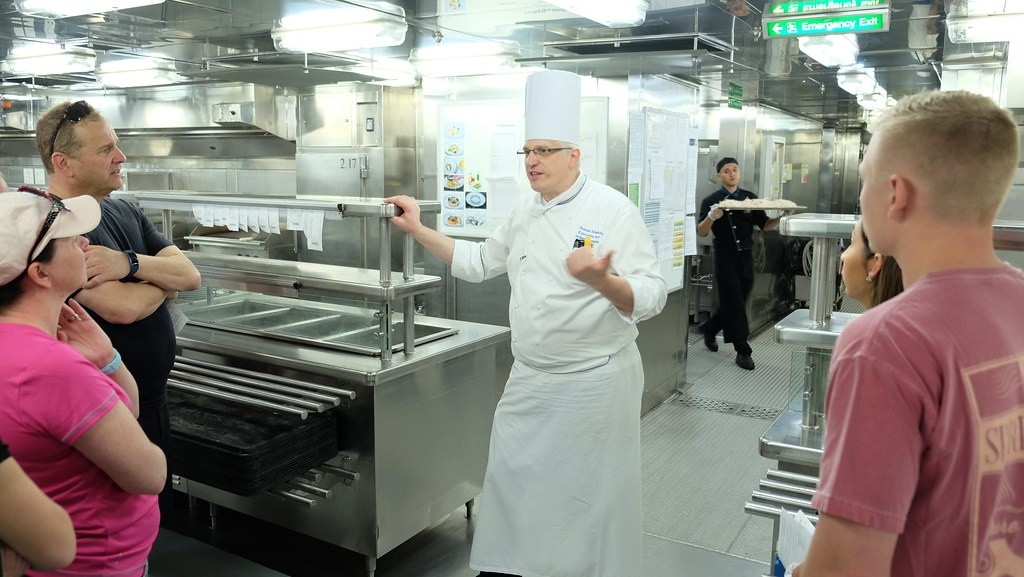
49 100 95 157
517 146 573 160
16 185 65 269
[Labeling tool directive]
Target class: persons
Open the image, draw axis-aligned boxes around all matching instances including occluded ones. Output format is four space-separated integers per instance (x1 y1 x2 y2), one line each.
695 157 794 370
0 185 168 577
0 440 76 577
0 172 9 193
839 218 903 310
33 100 201 451
385 70 668 577
788 90 1024 577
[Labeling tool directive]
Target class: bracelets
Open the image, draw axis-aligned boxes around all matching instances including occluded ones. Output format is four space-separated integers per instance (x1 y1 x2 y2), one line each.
123 250 140 281
100 348 121 377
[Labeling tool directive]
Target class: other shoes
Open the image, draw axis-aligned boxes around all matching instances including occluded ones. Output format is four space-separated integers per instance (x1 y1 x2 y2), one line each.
698 320 718 351
735 352 755 370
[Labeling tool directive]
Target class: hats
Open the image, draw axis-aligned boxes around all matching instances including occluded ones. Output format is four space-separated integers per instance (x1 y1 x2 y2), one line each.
524 70 581 147
717 158 739 174
0 191 102 287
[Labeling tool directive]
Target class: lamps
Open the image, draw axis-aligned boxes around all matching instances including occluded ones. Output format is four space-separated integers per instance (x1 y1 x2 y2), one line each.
541 0 651 32
799 34 897 110
945 14 1024 44
269 18 409 54
5 51 100 79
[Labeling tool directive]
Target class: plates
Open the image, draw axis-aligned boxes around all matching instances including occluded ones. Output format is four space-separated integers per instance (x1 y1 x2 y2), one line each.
443 213 463 226
444 177 464 189
444 194 463 209
444 158 458 173
466 193 486 207
445 143 462 155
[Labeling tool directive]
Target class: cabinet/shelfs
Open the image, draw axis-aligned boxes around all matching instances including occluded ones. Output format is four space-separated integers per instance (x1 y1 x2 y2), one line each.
110 189 444 364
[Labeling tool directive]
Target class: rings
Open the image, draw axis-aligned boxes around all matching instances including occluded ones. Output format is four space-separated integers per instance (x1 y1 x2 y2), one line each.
68 314 80 322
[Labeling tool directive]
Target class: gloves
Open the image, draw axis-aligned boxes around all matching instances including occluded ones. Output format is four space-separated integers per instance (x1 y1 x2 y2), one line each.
783 202 798 216
708 203 723 222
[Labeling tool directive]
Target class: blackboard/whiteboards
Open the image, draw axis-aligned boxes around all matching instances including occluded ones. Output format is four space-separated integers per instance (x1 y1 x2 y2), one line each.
640 106 690 294
